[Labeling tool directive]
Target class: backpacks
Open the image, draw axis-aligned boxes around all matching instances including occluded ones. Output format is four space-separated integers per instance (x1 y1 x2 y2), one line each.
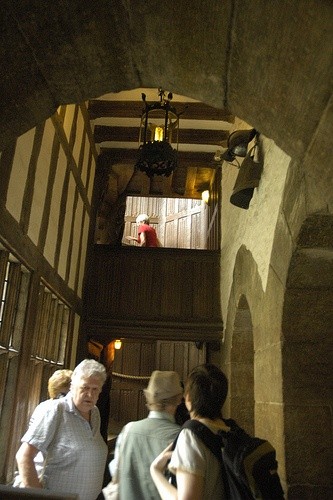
169 417 286 500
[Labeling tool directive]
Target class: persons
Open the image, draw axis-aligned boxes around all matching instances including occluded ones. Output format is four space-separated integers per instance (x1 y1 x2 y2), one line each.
150 364 232 500
125 214 160 247
103 370 190 500
15 359 108 500
13 370 73 488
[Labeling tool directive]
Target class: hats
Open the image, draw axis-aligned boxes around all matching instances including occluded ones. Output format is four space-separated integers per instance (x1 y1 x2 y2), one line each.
144 371 182 404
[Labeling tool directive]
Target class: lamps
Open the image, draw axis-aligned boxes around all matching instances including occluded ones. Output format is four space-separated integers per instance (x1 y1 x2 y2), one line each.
134 87 183 179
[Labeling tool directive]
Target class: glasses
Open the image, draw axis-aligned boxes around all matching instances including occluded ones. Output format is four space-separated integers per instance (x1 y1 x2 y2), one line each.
52 393 65 399
